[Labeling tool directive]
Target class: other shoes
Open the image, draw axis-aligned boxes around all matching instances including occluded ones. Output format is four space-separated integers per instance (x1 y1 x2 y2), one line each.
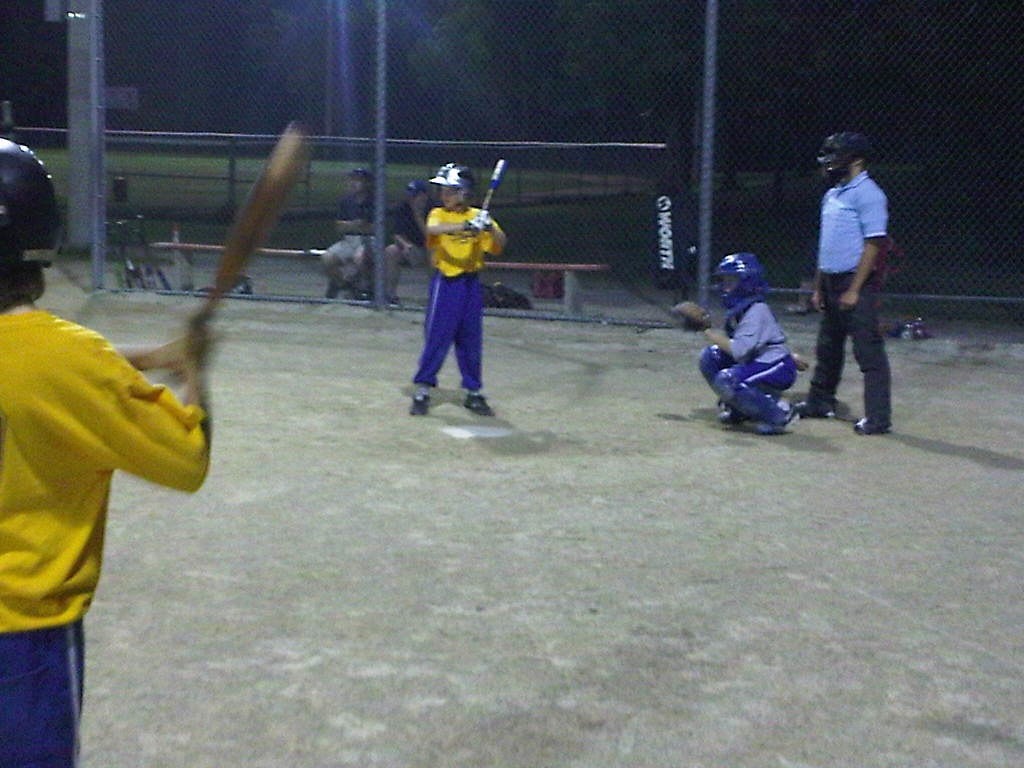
757 409 799 435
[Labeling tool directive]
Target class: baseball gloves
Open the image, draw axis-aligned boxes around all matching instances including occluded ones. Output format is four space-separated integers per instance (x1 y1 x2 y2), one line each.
676 301 712 333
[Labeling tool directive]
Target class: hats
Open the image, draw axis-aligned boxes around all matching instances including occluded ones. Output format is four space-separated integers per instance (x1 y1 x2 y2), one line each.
347 169 369 183
405 180 427 197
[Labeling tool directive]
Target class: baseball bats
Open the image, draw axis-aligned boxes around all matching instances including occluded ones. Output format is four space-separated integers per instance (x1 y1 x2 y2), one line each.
473 159 509 233
167 121 310 388
104 213 180 291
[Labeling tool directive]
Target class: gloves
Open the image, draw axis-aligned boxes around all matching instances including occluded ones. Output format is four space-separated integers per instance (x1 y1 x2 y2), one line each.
465 211 492 233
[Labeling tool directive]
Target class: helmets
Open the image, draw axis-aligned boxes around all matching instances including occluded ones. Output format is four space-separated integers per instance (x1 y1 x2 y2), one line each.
838 131 868 163
0 139 61 310
427 164 474 189
713 252 764 307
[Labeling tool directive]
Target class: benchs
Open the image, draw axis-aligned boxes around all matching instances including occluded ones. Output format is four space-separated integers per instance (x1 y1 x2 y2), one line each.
484 261 612 316
150 242 326 293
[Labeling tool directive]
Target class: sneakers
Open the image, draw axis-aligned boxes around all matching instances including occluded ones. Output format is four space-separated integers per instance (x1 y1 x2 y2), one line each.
857 415 891 433
791 398 834 416
409 392 427 416
719 408 752 425
463 395 495 416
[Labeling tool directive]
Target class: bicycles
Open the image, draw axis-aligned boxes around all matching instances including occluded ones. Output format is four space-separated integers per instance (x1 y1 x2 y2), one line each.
104 215 172 289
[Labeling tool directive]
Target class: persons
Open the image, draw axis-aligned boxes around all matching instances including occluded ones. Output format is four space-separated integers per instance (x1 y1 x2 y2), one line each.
320 166 442 309
411 164 504 418
672 253 801 436
789 130 893 437
0 135 217 767
